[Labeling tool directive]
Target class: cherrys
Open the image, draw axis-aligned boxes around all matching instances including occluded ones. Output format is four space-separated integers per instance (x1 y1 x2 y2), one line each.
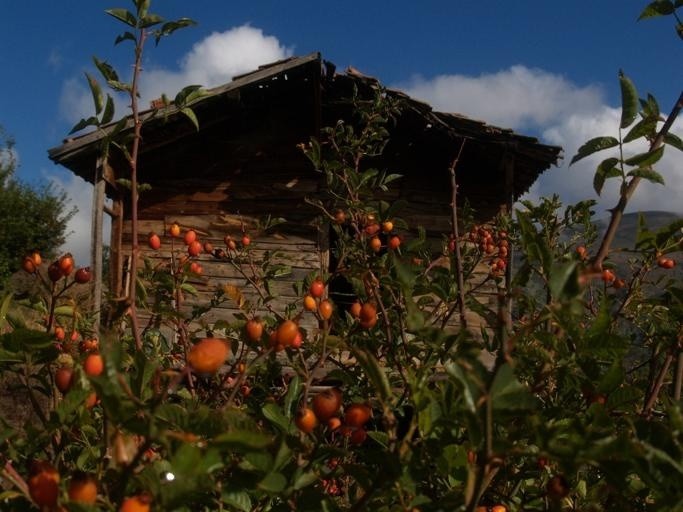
24 212 675 512
